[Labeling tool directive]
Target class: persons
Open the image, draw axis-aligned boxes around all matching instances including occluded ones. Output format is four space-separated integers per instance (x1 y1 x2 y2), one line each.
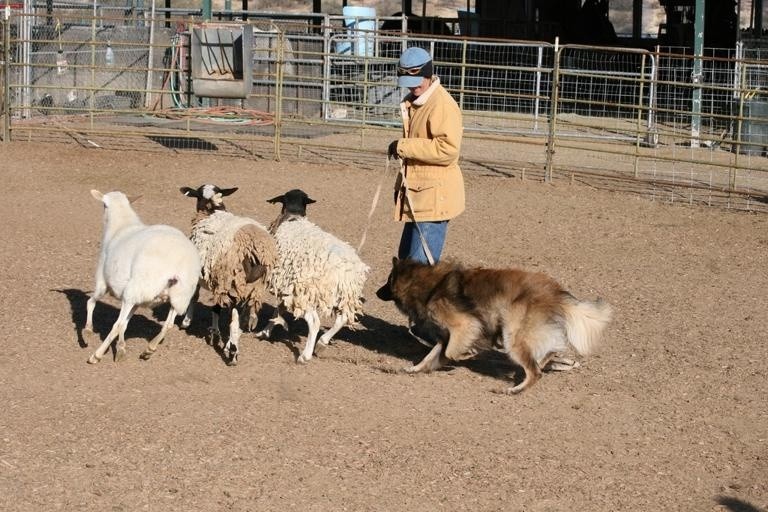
384 46 467 267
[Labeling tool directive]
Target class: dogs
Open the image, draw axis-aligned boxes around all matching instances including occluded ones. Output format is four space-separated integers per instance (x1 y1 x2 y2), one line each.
375 256 614 397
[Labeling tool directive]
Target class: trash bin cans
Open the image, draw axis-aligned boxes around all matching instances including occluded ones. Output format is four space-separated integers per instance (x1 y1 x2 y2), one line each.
731 98 768 157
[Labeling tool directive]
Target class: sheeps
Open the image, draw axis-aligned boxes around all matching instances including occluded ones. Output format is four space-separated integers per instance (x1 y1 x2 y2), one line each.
81 189 202 365
178 183 279 366
240 189 372 365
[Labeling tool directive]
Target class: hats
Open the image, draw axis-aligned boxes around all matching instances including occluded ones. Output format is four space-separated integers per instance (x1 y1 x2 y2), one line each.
396 46 432 88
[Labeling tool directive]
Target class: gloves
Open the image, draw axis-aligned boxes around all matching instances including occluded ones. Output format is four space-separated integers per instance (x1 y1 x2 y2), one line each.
387 141 397 161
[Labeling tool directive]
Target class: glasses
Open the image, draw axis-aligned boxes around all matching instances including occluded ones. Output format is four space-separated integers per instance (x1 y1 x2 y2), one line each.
397 63 427 75
